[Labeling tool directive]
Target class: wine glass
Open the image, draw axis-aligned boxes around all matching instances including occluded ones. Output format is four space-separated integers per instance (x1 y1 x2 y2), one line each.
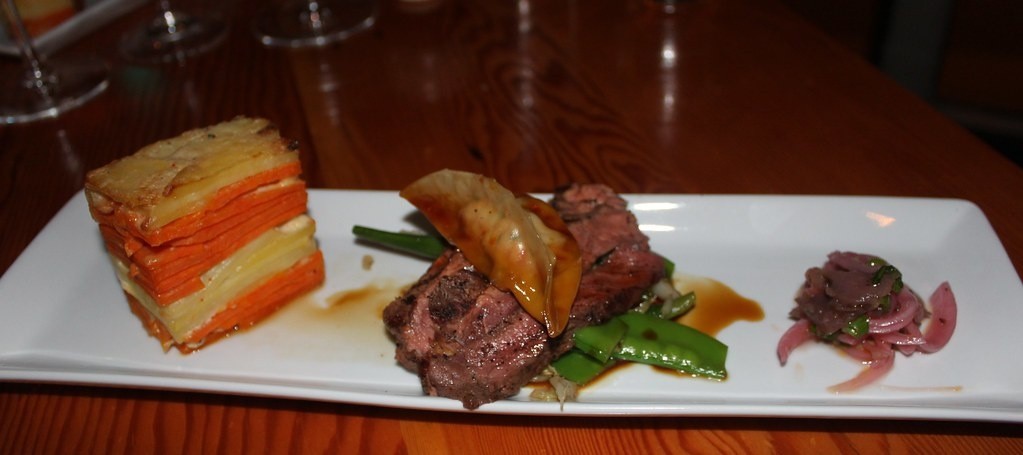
262 0 374 51
119 0 225 68
0 0 108 125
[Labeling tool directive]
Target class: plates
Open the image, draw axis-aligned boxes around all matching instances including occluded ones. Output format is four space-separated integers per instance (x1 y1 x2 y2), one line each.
0 0 143 56
0 187 1022 423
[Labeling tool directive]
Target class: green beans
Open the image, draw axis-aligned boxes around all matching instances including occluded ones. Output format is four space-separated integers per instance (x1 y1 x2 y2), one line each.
351 225 731 386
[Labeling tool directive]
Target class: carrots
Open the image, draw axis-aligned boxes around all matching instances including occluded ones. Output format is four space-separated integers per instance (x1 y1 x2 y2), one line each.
88 159 324 354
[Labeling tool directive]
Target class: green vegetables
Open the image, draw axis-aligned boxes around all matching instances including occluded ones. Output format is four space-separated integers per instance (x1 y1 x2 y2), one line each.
811 257 904 343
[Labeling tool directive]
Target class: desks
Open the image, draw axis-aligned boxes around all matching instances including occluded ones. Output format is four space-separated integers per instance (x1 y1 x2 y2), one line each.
0 0 1023 455
